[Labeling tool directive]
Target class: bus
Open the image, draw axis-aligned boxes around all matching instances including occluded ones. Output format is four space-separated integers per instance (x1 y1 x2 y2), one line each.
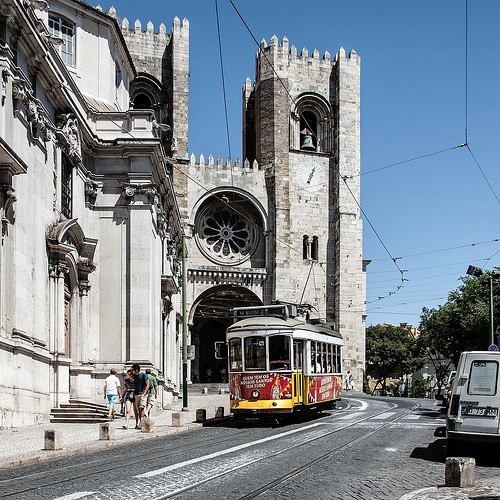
214 300 344 425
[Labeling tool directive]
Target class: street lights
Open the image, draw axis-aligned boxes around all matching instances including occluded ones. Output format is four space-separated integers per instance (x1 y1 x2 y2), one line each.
465 264 500 343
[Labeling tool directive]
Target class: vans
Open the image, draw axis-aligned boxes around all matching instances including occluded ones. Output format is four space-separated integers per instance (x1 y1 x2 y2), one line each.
446 350 500 442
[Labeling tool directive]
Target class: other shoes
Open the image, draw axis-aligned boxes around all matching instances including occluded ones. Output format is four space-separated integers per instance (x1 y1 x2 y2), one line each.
144 413 147 417
122 424 128 429
135 425 142 429
108 415 114 422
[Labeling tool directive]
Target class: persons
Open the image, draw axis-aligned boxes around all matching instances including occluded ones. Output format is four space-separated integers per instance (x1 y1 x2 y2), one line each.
207 369 211 383
310 356 332 373
220 367 226 382
345 371 354 392
103 369 122 420
121 364 157 431
